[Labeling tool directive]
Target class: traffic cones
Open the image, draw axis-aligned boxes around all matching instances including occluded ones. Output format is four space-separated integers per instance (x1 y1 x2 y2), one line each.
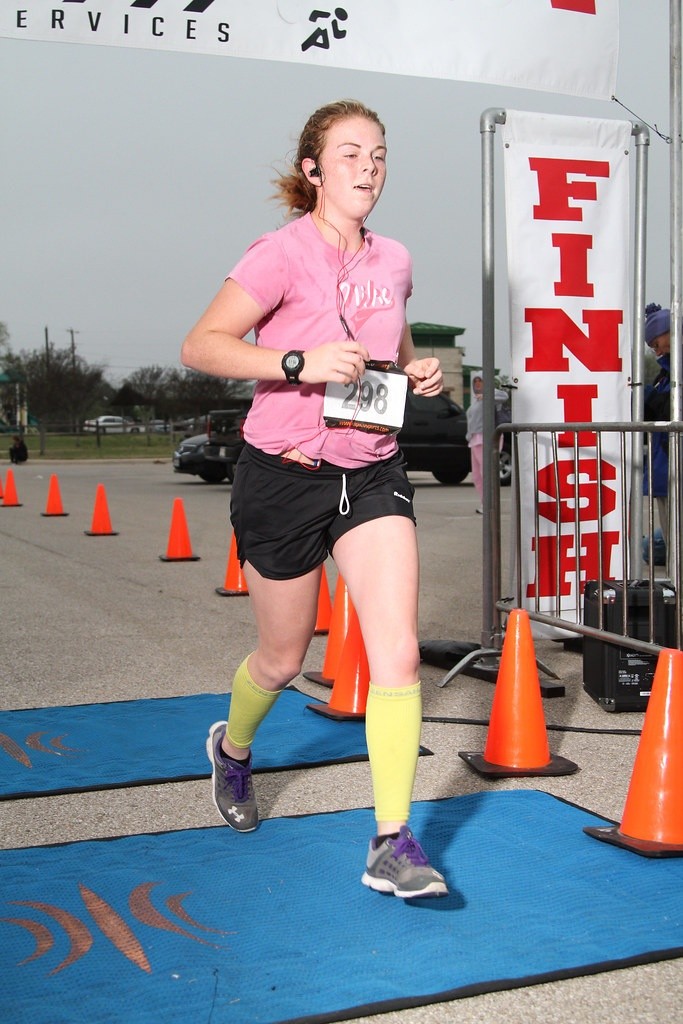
302 568 352 691
312 561 334 636
157 497 203 562
581 648 683 858
0 476 4 499
455 608 580 780
214 530 251 597
303 598 372 723
40 472 71 518
83 483 119 537
0 468 25 507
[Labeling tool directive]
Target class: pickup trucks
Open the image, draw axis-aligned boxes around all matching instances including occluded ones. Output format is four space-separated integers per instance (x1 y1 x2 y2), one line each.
206 372 474 487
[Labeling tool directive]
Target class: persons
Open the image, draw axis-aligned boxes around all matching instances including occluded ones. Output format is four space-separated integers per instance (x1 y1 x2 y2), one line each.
9 435 28 465
465 371 509 514
642 302 683 563
180 101 453 899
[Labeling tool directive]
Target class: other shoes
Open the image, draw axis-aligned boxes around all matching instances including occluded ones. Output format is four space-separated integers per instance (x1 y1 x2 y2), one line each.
476 507 483 514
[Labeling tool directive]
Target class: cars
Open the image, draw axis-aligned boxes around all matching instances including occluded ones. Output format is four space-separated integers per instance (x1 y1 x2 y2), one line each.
171 433 229 484
149 419 170 432
82 416 145 434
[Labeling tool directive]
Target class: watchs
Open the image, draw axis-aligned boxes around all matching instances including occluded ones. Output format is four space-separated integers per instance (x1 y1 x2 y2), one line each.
282 349 305 385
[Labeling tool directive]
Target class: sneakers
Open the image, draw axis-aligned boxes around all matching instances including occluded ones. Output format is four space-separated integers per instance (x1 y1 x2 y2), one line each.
205 721 258 832
360 825 449 900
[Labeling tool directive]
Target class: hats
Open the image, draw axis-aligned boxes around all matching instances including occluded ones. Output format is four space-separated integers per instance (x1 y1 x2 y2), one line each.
644 302 670 346
